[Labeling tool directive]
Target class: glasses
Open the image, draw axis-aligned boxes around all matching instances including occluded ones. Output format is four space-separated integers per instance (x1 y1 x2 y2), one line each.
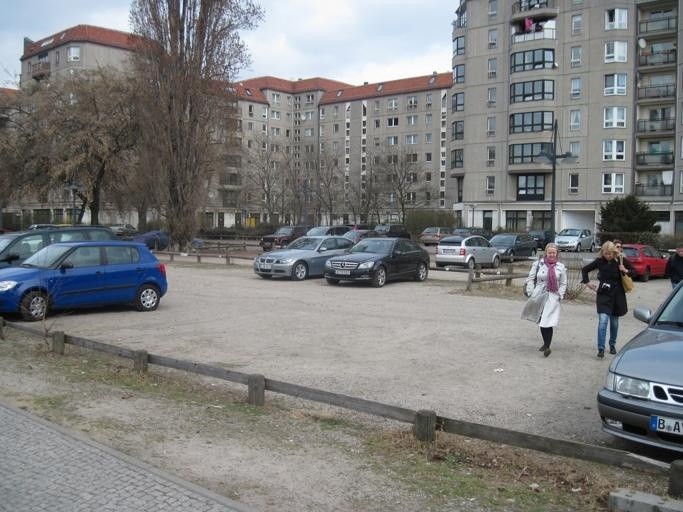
616 245 622 248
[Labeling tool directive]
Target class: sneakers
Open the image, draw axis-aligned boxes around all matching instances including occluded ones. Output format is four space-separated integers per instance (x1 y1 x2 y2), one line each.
544 349 552 357
539 344 545 351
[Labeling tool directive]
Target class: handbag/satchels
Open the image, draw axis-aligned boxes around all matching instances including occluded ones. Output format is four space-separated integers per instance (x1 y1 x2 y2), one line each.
522 260 540 298
619 253 634 293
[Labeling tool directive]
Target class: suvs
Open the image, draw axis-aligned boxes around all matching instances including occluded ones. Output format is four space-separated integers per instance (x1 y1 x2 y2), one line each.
258 224 308 252
0 221 118 270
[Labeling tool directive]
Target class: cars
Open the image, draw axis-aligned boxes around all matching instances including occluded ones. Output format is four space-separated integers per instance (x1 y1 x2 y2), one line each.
322 235 432 288
107 221 138 235
656 247 677 261
622 243 669 282
303 220 559 271
252 235 357 282
595 276 683 456
0 239 170 323
553 226 596 254
129 229 205 251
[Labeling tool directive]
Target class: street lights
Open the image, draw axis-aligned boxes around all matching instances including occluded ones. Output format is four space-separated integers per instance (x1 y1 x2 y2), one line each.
469 203 478 227
533 151 581 244
64 182 78 225
243 208 248 228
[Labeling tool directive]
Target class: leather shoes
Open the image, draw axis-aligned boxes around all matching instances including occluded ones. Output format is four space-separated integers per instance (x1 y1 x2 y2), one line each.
610 345 616 354
598 349 604 357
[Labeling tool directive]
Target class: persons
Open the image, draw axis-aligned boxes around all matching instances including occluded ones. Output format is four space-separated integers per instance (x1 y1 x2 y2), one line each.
519 242 567 356
515 24 542 35
665 243 682 290
611 238 630 262
580 240 636 357
